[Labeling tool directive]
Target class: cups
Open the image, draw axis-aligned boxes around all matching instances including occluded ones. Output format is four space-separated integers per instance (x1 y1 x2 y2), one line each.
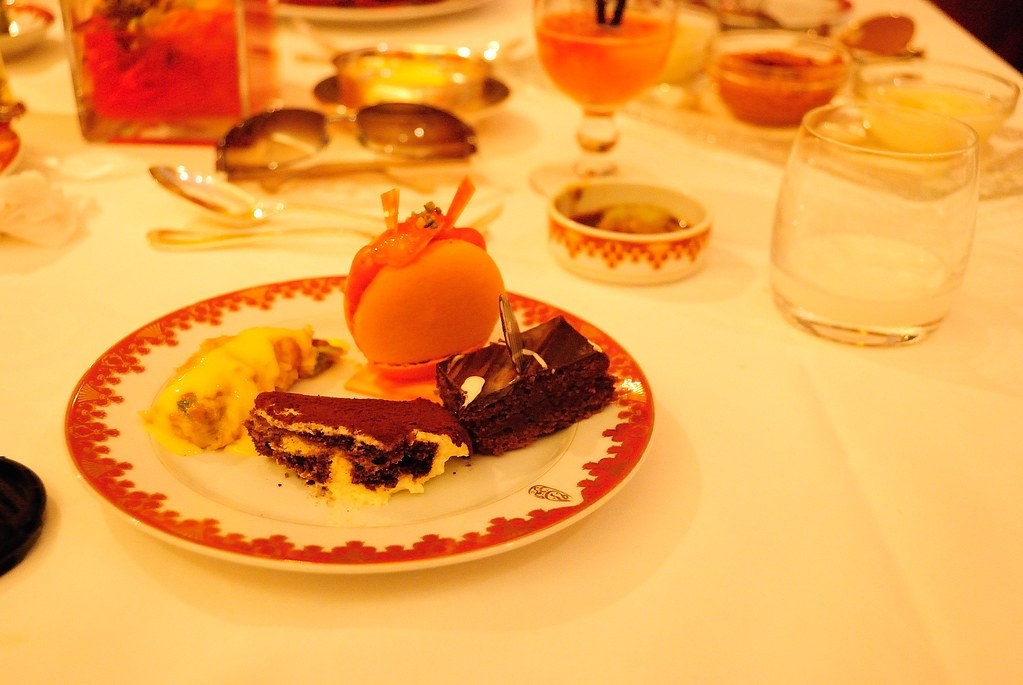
768 103 977 351
854 58 1021 154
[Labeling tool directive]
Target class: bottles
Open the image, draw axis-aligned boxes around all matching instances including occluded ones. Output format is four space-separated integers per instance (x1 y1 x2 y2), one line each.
61 0 278 148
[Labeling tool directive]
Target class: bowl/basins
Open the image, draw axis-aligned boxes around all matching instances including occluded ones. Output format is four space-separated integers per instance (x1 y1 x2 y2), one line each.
703 28 855 129
331 43 488 113
0 3 55 59
547 176 711 287
660 4 718 85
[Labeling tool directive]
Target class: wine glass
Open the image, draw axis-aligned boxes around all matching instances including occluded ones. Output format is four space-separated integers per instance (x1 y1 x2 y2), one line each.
528 0 686 197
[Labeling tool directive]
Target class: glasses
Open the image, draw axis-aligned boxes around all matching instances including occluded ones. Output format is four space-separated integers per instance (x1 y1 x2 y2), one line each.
215 99 480 187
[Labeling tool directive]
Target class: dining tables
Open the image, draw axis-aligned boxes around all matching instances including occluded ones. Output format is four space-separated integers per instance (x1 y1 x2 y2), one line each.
0 0 1023 685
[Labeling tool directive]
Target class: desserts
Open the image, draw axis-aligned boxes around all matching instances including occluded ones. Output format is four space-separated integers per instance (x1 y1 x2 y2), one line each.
151 177 615 498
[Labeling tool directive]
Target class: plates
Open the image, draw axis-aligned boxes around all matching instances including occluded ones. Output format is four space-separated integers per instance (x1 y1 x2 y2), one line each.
308 76 510 118
264 0 482 22
65 276 655 574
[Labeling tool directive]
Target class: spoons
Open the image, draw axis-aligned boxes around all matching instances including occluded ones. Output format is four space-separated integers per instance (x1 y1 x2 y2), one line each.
0 123 25 180
149 164 445 228
854 16 915 55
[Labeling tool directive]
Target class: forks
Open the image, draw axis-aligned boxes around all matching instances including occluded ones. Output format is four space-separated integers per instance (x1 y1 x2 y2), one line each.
146 203 504 243
